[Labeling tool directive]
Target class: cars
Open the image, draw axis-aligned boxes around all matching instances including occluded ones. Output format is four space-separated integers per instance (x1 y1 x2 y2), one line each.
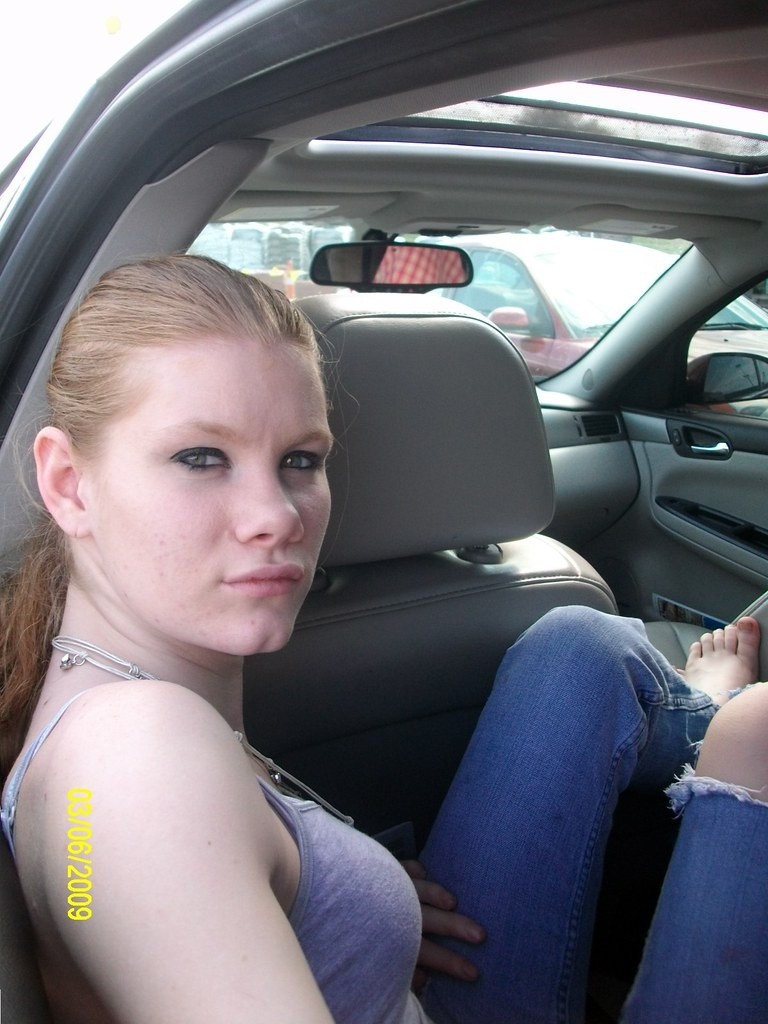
413 232 768 416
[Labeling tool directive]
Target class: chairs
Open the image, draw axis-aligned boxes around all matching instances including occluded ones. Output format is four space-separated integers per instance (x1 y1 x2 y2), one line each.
243 293 619 858
645 592 768 683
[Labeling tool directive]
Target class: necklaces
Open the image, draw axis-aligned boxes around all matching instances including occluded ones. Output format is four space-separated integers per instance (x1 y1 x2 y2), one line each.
51 635 356 826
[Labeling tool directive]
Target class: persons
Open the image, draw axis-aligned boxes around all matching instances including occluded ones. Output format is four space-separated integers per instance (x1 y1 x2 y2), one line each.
0 255 768 1024
